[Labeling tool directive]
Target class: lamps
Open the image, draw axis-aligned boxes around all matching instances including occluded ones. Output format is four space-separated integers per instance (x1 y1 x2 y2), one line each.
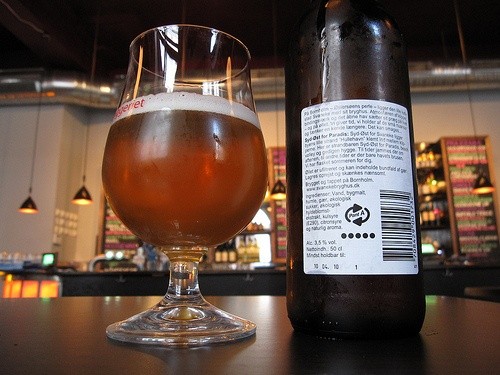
74 0 102 206
20 79 43 211
454 0 493 189
270 0 284 195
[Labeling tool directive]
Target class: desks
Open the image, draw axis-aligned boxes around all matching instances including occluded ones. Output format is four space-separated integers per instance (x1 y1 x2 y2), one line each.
0 298 500 375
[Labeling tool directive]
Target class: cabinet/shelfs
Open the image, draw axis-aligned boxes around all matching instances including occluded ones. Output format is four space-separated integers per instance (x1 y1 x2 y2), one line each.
94 134 500 269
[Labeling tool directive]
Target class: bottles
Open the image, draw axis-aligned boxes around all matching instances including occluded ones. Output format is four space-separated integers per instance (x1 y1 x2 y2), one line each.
284 0 427 350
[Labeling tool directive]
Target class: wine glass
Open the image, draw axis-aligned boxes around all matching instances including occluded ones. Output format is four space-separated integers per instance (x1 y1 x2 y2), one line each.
101 24 269 348
